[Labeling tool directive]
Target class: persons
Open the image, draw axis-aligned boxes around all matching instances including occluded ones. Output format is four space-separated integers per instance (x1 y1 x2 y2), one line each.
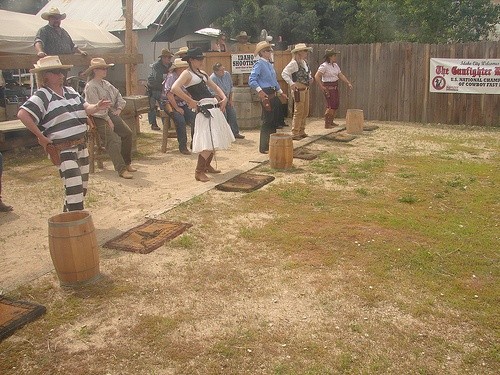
268 57 289 128
211 32 227 52
80 57 137 179
231 30 256 54
280 42 314 140
160 57 199 155
175 46 190 61
313 48 353 129
247 40 289 154
33 7 88 86
170 47 236 182
206 62 246 139
15 55 111 213
145 47 175 130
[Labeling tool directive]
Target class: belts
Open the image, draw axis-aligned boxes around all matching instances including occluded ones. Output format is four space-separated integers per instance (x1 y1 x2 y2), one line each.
203 103 220 108
327 86 338 90
50 135 87 149
299 87 310 91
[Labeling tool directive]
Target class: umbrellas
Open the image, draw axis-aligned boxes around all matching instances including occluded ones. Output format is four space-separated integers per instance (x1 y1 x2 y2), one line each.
151 0 241 45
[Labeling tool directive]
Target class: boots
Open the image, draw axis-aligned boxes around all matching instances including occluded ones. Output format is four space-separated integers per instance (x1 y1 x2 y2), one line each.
195 154 209 182
324 108 339 129
206 153 221 173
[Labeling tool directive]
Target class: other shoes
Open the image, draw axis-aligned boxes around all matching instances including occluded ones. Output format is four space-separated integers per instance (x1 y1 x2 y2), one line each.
0 196 13 212
292 133 308 140
119 169 133 178
151 125 160 130
126 162 137 171
236 134 245 139
180 145 191 155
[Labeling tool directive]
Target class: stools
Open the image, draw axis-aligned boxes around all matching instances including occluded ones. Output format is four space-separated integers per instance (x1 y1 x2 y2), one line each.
159 108 179 153
83 112 114 174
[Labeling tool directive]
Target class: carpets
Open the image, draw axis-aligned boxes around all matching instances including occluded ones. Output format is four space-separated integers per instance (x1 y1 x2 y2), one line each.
363 123 380 131
0 293 47 342
293 146 327 160
101 217 194 255
322 132 357 143
215 171 276 194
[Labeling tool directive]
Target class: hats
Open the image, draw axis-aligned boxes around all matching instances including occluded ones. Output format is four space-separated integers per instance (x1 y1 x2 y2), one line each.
41 8 67 20
29 55 74 73
254 41 276 57
323 48 335 58
291 42 313 54
181 48 204 61
236 31 252 40
158 49 173 57
171 58 189 69
83 57 115 76
174 47 188 55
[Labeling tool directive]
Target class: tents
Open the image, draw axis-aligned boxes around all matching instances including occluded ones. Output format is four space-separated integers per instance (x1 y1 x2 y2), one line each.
0 8 124 56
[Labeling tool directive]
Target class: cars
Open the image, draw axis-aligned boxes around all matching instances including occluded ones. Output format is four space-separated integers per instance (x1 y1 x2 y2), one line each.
5 80 36 99
